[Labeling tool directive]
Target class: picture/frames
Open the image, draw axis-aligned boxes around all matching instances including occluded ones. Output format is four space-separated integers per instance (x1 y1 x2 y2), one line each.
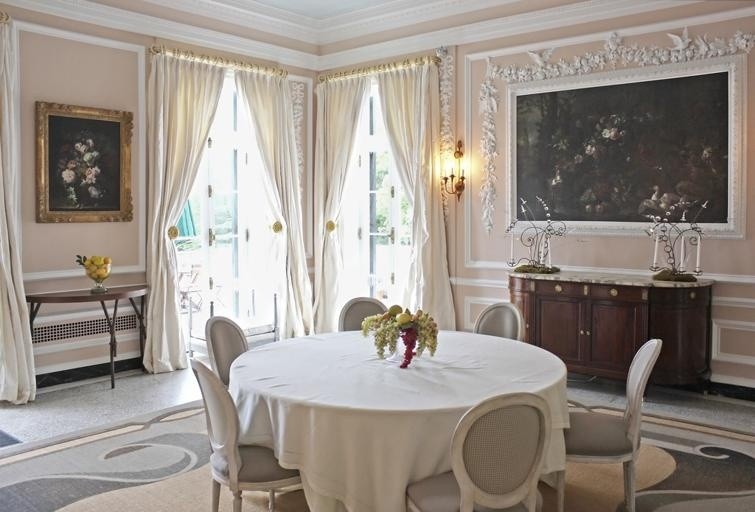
505 49 749 240
33 99 134 223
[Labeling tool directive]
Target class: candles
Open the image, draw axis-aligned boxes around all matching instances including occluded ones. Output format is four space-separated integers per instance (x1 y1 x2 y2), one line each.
695 235 702 272
539 233 546 263
651 234 660 269
547 236 554 272
509 228 515 264
678 235 686 272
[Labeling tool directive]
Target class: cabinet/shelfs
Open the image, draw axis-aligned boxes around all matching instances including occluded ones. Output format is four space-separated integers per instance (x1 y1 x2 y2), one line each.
510 269 715 393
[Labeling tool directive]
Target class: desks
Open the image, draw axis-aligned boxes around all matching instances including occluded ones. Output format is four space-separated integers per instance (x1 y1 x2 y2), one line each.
25 284 152 389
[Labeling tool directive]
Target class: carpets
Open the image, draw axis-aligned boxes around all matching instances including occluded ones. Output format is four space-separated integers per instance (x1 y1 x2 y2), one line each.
1 396 755 510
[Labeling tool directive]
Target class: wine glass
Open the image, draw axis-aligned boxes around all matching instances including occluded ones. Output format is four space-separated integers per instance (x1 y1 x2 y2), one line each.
86 265 111 293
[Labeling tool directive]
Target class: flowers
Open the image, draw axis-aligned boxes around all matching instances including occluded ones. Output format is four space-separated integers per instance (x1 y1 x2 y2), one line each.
360 304 438 369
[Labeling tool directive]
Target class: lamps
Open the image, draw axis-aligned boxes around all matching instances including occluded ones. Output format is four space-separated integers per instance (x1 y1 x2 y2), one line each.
442 136 470 202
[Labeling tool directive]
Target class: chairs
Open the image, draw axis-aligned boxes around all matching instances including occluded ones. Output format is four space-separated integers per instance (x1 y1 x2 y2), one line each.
405 392 554 511
189 359 306 510
204 315 249 388
473 301 525 341
560 337 664 509
339 296 392 332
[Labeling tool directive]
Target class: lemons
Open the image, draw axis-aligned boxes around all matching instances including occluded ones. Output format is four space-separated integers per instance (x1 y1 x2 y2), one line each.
84 256 111 279
389 305 410 325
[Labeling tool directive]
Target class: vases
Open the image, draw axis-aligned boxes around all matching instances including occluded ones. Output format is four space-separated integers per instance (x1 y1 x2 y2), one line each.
394 331 414 361
